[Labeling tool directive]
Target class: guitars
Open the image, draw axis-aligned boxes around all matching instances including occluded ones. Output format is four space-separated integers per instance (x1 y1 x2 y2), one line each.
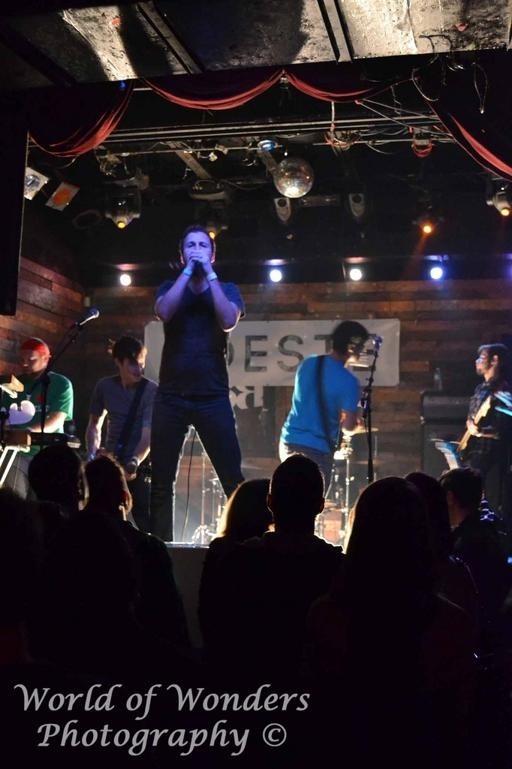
435 395 491 470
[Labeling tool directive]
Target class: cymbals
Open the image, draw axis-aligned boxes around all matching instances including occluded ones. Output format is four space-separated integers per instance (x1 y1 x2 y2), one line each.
343 425 378 434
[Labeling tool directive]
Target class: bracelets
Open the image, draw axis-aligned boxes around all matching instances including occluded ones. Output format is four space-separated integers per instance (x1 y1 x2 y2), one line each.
183 267 193 276
207 271 217 281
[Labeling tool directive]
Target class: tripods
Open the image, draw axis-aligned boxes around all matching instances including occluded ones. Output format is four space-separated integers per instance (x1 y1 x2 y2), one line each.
190 452 216 545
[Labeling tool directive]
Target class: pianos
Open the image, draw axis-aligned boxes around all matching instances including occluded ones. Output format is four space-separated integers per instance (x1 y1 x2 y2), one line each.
1 429 81 448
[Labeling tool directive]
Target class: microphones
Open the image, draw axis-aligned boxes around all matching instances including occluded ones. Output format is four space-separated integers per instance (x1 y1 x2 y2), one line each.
373 336 383 357
74 308 99 327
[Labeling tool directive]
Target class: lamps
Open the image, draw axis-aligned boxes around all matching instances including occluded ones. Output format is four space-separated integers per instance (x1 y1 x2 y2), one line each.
271 146 316 200
104 178 143 228
482 176 512 223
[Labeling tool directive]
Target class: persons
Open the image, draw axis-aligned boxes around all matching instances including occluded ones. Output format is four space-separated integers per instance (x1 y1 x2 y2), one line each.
143 223 247 539
275 320 372 502
81 336 160 539
449 340 511 488
0 441 512 764
0 337 74 499
